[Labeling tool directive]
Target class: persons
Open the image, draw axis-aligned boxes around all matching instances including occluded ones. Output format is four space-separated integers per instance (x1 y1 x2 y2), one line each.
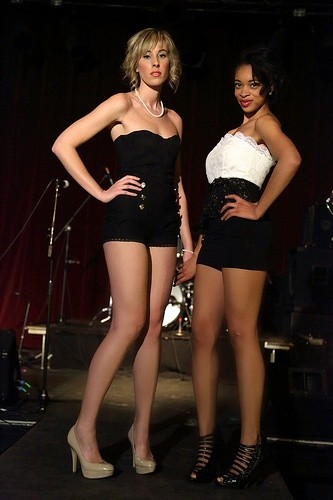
175 50 301 488
52 27 193 479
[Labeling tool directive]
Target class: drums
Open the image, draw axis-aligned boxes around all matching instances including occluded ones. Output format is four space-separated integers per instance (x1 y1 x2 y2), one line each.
162 281 186 327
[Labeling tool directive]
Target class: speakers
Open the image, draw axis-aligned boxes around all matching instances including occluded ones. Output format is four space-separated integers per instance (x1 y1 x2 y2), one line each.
0 330 22 407
269 201 333 439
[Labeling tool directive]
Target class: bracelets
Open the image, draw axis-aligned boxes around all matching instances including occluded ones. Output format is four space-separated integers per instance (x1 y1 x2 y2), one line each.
181 249 194 254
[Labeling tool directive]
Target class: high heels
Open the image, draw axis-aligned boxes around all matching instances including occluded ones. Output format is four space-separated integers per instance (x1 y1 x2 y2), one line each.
128 425 156 475
188 431 224 485
66 426 114 479
217 435 270 491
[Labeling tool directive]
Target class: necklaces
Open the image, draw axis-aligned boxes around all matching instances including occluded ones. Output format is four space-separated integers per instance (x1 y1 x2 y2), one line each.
135 88 164 117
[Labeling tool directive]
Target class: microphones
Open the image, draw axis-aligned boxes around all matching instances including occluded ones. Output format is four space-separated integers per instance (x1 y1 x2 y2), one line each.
103 161 114 186
56 180 70 189
